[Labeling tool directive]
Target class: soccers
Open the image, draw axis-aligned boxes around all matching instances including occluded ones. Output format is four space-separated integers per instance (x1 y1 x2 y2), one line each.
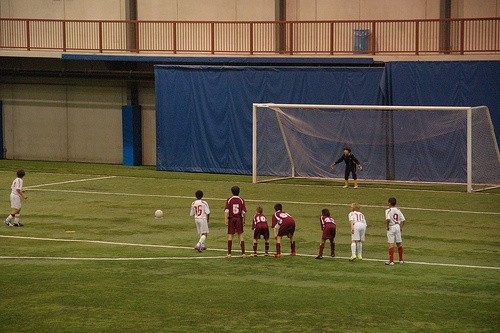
155 210 164 218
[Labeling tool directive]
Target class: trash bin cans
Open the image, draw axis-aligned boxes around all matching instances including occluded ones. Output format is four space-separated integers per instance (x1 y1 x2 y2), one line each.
353 29 369 54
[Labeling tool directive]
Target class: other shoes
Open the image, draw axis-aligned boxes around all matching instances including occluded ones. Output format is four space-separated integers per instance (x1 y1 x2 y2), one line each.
331 254 335 257
265 253 268 256
275 254 280 257
385 261 395 265
228 254 231 257
195 246 202 252
201 247 207 250
254 252 257 255
399 261 403 263
354 186 358 189
290 253 295 256
242 254 246 258
343 186 348 188
349 256 356 261
357 257 362 259
316 256 322 259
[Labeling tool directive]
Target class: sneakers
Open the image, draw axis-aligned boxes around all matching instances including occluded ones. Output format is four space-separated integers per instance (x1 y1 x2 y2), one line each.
13 222 23 227
4 220 13 226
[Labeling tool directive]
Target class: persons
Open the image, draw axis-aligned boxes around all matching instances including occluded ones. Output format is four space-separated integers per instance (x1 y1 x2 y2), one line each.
272 203 297 258
224 186 247 259
189 190 210 253
249 206 270 256
348 203 367 261
385 198 406 265
4 169 28 227
314 209 336 259
331 148 362 189
